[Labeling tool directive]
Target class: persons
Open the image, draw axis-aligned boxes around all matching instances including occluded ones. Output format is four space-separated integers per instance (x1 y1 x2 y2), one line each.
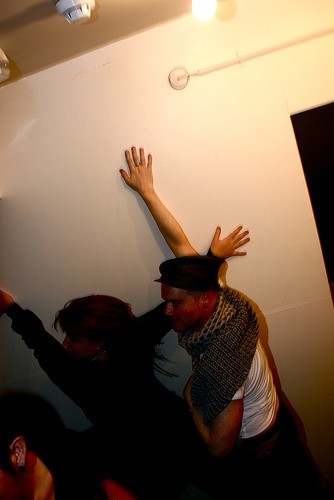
0 409 141 500
0 226 251 500
118 145 334 500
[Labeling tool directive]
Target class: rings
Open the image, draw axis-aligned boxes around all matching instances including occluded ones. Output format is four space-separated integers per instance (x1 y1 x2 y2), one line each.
134 161 141 166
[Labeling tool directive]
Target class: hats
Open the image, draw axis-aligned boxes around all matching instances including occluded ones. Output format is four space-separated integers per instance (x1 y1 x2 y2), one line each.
154 256 223 292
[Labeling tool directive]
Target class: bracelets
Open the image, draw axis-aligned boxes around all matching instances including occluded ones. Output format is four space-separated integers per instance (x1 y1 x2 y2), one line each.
189 407 195 412
